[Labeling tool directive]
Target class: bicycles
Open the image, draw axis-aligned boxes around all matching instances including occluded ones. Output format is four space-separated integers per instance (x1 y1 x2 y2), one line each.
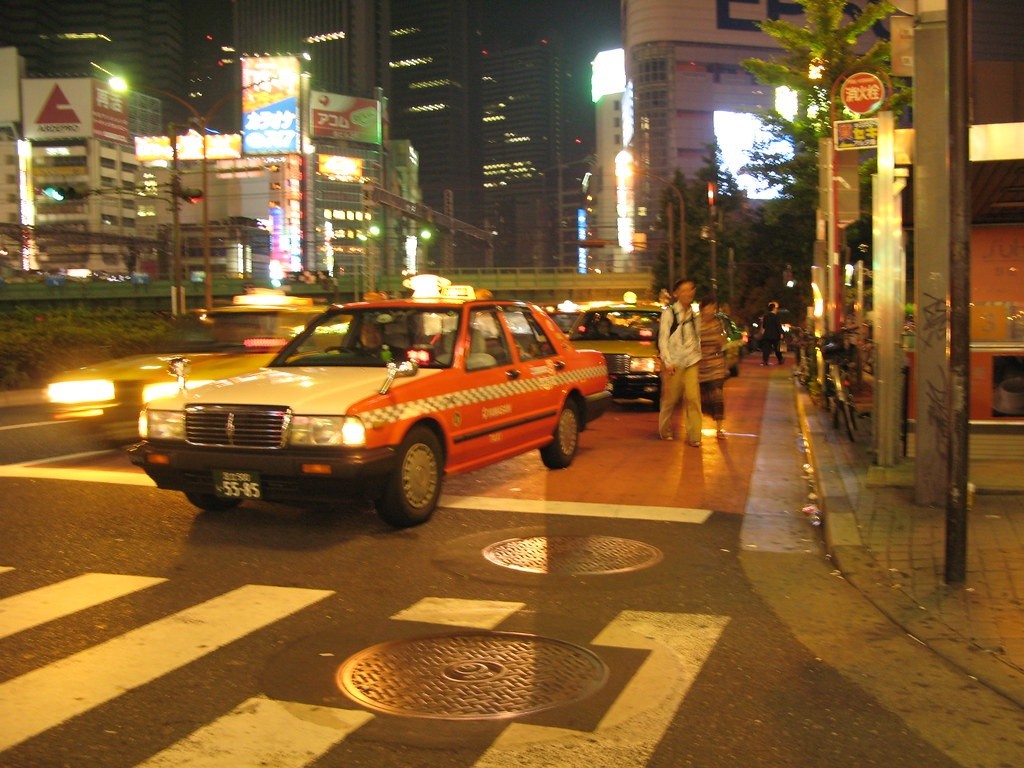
802 322 868 445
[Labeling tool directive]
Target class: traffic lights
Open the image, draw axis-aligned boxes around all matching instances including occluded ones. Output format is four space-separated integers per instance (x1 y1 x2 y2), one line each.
40 184 83 202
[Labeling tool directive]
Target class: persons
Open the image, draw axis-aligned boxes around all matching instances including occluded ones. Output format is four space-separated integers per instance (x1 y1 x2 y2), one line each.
759 302 785 367
593 318 621 340
658 278 730 446
355 312 406 361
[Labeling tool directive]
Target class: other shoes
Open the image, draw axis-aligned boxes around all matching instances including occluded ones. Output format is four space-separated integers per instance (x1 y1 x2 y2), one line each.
662 436 673 440
717 430 728 439
690 441 702 446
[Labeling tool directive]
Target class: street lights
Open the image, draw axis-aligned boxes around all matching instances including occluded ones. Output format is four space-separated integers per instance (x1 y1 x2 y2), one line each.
107 68 298 311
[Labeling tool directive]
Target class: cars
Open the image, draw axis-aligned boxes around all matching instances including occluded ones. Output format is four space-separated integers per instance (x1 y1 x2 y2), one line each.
128 296 615 530
711 303 755 378
567 302 662 407
